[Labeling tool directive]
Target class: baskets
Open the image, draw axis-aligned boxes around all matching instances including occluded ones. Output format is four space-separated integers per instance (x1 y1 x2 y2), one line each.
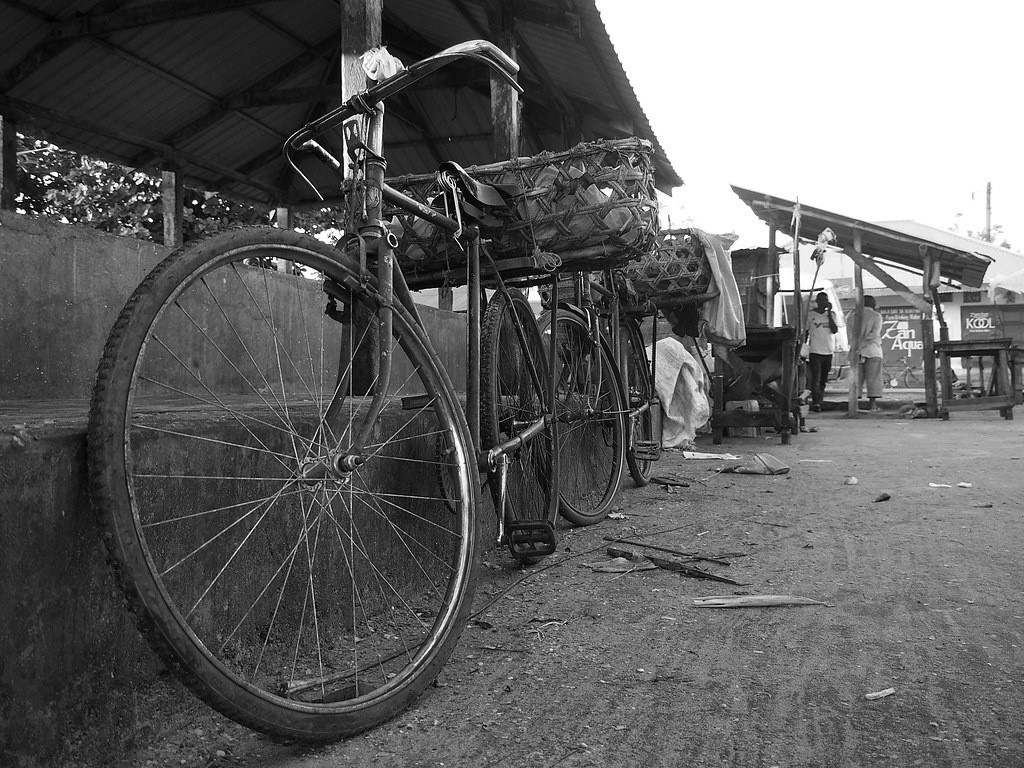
540 228 710 312
389 137 658 284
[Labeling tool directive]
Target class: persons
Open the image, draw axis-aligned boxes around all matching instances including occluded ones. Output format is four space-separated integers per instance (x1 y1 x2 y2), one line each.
804 292 838 412
846 295 884 412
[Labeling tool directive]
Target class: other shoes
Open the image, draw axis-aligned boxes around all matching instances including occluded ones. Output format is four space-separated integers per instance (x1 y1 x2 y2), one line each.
812 403 821 412
871 408 882 413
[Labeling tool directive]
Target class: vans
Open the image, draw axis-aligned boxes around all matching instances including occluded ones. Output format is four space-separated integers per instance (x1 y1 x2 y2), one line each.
774 279 850 380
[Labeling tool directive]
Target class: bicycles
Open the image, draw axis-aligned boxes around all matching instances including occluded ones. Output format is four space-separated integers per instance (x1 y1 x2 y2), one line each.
86 39 721 745
883 356 924 389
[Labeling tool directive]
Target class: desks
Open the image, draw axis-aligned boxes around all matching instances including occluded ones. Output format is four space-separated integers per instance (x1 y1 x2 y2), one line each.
707 324 799 446
933 337 1015 420
